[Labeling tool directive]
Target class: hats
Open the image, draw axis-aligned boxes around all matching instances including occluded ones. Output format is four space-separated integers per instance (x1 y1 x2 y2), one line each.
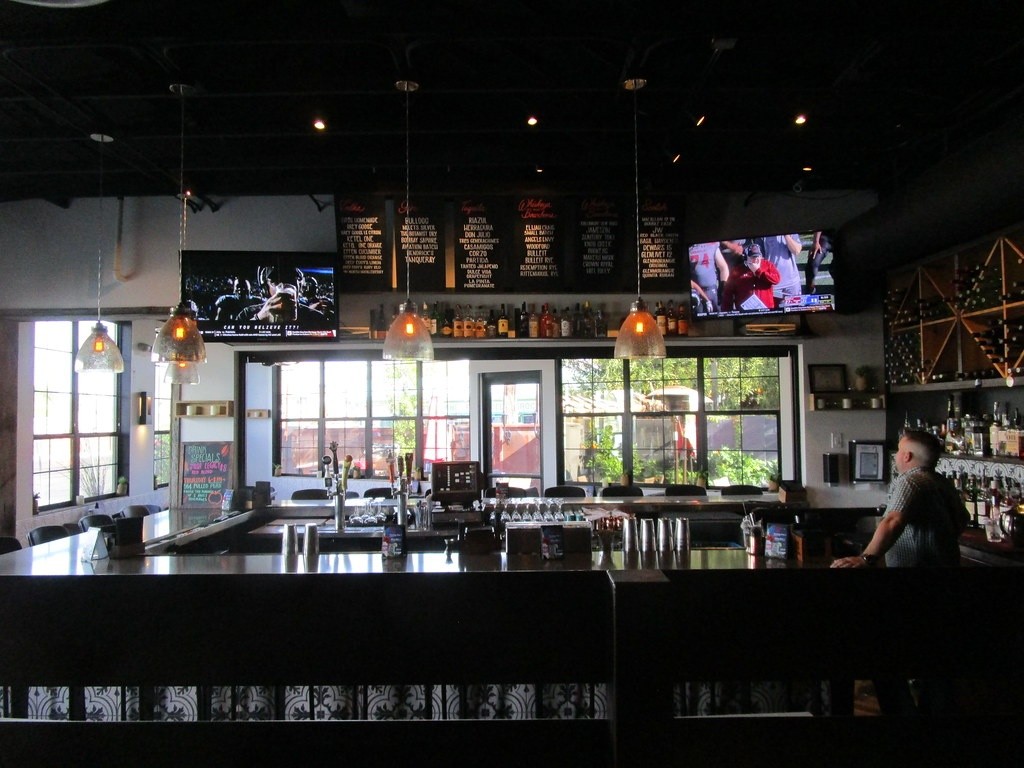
745 244 761 257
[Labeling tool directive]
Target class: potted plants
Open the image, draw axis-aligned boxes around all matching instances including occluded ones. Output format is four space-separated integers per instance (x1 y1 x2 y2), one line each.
32 493 41 514
117 478 128 497
854 365 871 389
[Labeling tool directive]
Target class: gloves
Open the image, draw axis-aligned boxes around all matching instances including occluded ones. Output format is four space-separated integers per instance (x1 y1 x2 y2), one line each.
706 301 713 313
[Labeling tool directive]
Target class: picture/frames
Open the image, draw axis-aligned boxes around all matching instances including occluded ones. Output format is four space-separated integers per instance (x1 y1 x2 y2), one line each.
847 440 886 478
808 363 849 391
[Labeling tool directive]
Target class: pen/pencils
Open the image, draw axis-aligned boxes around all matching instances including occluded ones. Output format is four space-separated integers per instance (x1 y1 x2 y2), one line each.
742 512 756 526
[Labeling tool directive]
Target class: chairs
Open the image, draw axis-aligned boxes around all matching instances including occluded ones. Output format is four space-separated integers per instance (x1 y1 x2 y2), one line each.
292 489 328 500
0 537 21 555
600 486 643 497
664 484 706 497
121 504 149 518
79 515 112 532
721 484 763 495
486 488 527 498
544 486 585 496
25 524 72 546
362 488 399 499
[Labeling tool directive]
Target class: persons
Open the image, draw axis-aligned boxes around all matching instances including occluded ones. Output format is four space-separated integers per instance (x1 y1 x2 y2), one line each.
829 432 971 568
688 231 831 312
186 265 335 322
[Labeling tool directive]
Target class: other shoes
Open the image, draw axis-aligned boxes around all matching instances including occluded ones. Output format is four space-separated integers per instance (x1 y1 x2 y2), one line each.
805 287 816 294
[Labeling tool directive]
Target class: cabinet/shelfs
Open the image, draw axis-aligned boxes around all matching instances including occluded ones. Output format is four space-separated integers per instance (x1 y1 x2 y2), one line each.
889 379 1024 563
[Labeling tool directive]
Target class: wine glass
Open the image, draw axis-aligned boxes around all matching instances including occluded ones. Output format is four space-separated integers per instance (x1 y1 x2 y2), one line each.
349 496 387 526
490 498 564 527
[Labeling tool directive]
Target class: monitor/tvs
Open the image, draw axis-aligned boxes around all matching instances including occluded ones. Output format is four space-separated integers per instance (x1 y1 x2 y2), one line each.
178 249 340 341
685 229 848 323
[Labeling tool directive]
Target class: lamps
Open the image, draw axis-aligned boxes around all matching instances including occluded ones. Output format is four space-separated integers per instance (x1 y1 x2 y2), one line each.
140 391 146 424
381 74 437 363
614 79 668 362
153 81 206 366
74 133 127 376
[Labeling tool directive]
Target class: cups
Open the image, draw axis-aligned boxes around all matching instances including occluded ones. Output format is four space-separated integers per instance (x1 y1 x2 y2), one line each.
745 532 764 554
76 496 84 505
871 399 882 408
640 518 656 552
657 518 674 552
420 481 430 494
282 522 298 558
843 398 852 408
302 523 319 556
412 481 418 494
675 517 690 552
984 519 1001 542
816 399 825 409
623 516 638 552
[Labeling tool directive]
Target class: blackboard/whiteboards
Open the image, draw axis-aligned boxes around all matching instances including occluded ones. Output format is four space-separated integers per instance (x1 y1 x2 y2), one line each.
178 440 234 509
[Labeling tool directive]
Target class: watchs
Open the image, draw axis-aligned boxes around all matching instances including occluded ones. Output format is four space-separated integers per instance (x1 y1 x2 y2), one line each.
860 554 876 567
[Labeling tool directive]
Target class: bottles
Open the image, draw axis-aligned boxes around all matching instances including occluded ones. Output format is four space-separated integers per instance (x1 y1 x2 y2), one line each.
652 299 688 337
369 299 609 338
898 395 1024 539
884 256 1024 386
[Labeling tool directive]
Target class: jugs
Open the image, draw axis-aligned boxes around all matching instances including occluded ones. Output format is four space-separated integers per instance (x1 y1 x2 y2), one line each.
999 504 1024 547
